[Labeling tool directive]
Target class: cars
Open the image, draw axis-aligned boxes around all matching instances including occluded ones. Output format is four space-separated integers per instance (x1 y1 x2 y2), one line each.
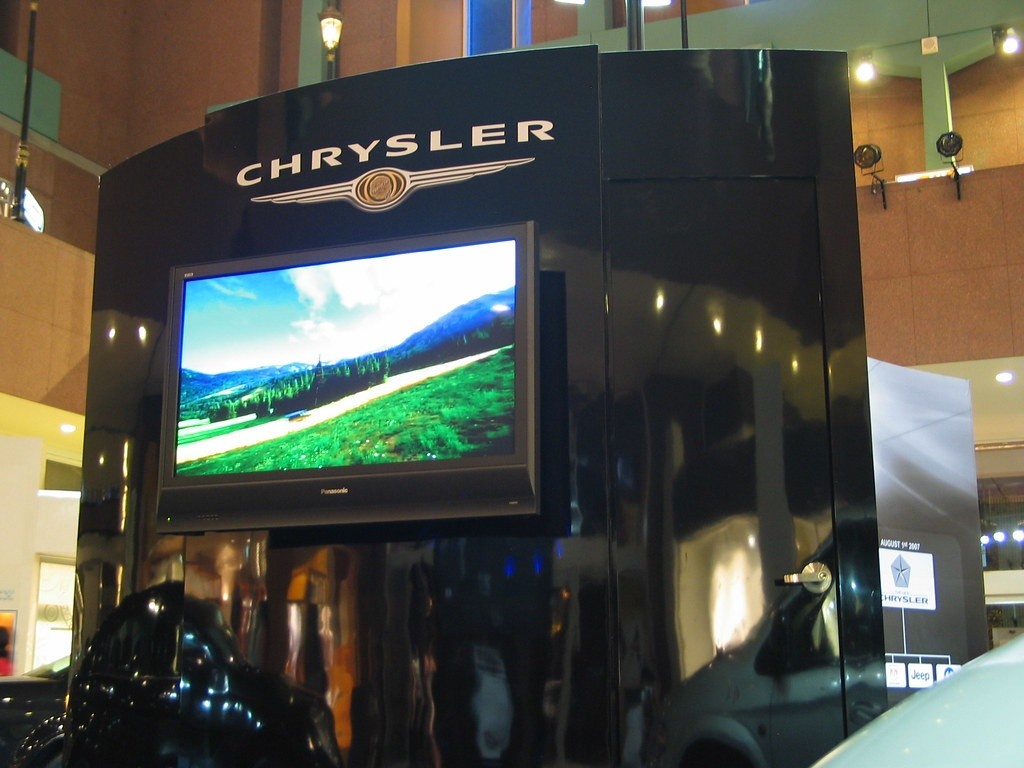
0 658 76 768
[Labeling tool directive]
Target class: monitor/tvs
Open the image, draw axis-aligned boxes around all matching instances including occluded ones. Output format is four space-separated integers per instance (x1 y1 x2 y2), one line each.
151 216 547 536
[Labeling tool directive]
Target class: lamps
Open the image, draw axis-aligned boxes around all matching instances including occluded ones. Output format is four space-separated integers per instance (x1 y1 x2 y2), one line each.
935 132 963 200
990 28 1022 54
853 143 887 209
